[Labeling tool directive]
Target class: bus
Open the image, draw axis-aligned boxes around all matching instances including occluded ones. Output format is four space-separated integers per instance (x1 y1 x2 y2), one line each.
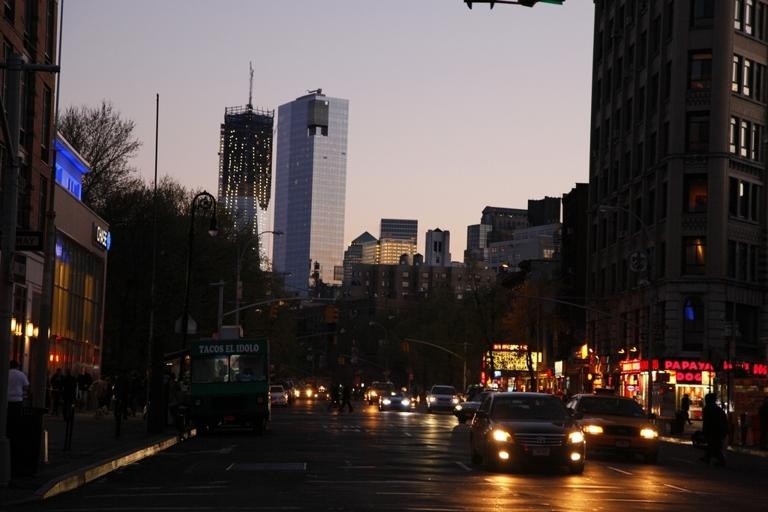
182 332 273 438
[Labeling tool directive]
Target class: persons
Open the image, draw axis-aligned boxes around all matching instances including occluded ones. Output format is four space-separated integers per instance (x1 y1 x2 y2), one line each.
325 380 354 413
692 392 731 464
6 359 31 436
46 366 179 419
681 393 694 425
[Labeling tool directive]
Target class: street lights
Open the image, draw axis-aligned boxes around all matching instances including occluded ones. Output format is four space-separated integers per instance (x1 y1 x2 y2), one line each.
166 191 223 431
597 202 656 414
366 322 387 334
234 227 285 327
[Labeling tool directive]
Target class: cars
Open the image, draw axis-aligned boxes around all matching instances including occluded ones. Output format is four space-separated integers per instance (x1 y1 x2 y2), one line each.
263 371 503 424
561 390 661 462
458 389 589 477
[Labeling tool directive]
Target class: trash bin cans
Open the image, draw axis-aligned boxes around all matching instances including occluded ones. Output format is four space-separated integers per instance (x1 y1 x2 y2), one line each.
6 406 49 480
